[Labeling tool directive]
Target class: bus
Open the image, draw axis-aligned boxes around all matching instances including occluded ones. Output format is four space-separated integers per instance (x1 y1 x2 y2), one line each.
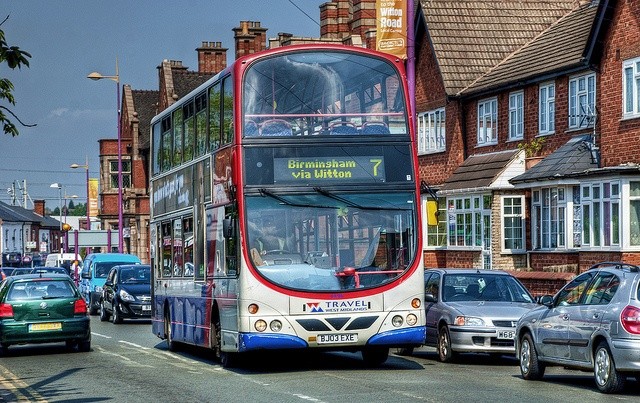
149 44 440 368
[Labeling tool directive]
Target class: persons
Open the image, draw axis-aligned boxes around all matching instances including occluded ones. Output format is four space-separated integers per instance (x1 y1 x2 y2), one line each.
47 284 59 299
174 248 194 275
250 218 295 255
429 283 440 301
213 150 232 206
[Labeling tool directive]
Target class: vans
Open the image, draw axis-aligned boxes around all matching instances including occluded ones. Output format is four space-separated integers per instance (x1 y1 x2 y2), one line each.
45 253 83 274
79 253 142 315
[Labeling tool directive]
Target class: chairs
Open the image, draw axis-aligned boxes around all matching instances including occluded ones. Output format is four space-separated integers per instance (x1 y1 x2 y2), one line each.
49 288 64 295
330 121 357 135
362 120 388 135
260 119 293 137
444 286 456 302
466 284 479 297
11 289 28 297
229 119 259 142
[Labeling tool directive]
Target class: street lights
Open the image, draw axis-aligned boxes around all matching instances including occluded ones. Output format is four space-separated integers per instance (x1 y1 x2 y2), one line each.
70 163 90 254
49 182 64 266
64 194 78 253
86 71 123 253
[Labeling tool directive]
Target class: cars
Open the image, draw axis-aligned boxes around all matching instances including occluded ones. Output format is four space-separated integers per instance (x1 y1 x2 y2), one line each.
30 266 68 274
100 264 152 323
397 268 542 362
0 273 90 351
10 268 34 276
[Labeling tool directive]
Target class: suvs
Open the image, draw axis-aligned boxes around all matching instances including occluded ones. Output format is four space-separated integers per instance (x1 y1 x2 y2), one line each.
514 262 640 392
